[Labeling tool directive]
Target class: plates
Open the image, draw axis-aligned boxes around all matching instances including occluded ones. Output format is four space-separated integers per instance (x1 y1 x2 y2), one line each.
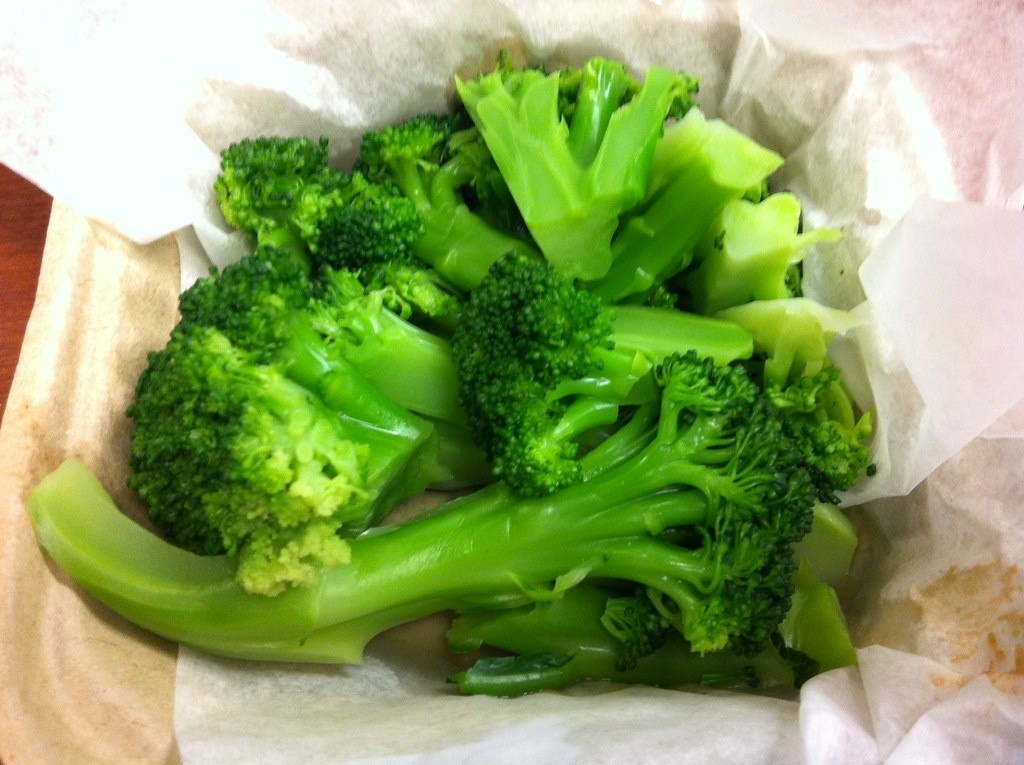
0 197 183 765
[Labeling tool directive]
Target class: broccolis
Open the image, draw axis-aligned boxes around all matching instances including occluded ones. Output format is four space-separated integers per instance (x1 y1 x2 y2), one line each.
26 50 875 698
